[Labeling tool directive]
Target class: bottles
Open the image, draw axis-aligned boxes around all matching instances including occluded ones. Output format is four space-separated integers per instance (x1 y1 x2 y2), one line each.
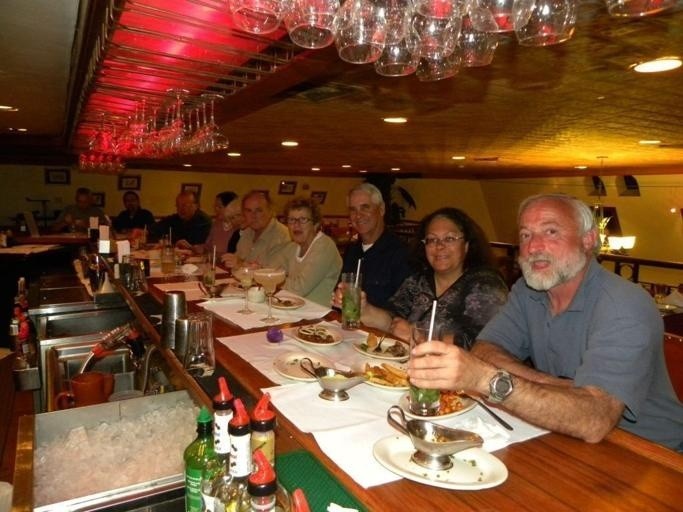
184 376 278 512
10 277 38 368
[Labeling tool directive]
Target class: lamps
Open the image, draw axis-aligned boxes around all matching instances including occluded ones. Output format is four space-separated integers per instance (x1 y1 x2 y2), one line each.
596 156 636 255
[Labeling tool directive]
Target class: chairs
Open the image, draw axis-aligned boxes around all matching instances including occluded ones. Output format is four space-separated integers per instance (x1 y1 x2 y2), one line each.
661 332 682 404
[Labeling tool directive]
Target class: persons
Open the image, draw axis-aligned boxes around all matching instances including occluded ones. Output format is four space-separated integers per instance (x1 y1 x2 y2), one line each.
223 197 249 254
488 374 520 407
177 191 238 258
335 207 509 353
48 187 111 235
146 191 212 244
221 189 291 281
333 182 416 313
401 193 682 455
243 196 343 310
111 190 155 235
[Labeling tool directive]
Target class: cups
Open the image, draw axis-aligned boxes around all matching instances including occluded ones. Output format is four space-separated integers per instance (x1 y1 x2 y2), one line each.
228 0 683 83
201 245 223 300
387 405 484 471
55 372 105 410
161 241 176 274
410 319 448 416
341 273 363 331
121 254 148 294
299 359 370 400
109 390 143 401
163 290 216 374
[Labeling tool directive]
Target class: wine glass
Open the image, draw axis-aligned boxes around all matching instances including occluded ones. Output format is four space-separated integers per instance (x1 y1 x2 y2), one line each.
79 89 230 172
253 266 286 323
231 263 260 315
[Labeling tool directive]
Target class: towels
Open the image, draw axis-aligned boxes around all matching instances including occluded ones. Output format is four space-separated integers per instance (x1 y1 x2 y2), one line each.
152 275 244 303
214 319 412 386
192 290 340 331
261 377 552 489
142 261 225 280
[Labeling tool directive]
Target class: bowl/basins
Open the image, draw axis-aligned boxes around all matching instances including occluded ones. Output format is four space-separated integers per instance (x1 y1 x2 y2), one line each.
248 286 267 302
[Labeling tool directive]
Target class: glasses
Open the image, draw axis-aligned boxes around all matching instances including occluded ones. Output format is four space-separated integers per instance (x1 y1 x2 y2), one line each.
286 216 315 224
422 235 463 244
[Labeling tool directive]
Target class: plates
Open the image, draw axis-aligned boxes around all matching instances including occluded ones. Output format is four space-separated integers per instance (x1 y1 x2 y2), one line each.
373 432 509 490
291 326 343 346
353 337 412 362
657 305 677 312
350 359 411 389
266 296 305 309
273 350 335 381
398 389 482 421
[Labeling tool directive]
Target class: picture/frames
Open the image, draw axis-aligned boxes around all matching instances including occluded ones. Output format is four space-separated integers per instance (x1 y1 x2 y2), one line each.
45 169 70 184
311 191 327 204
182 183 202 195
279 181 297 194
118 175 142 191
